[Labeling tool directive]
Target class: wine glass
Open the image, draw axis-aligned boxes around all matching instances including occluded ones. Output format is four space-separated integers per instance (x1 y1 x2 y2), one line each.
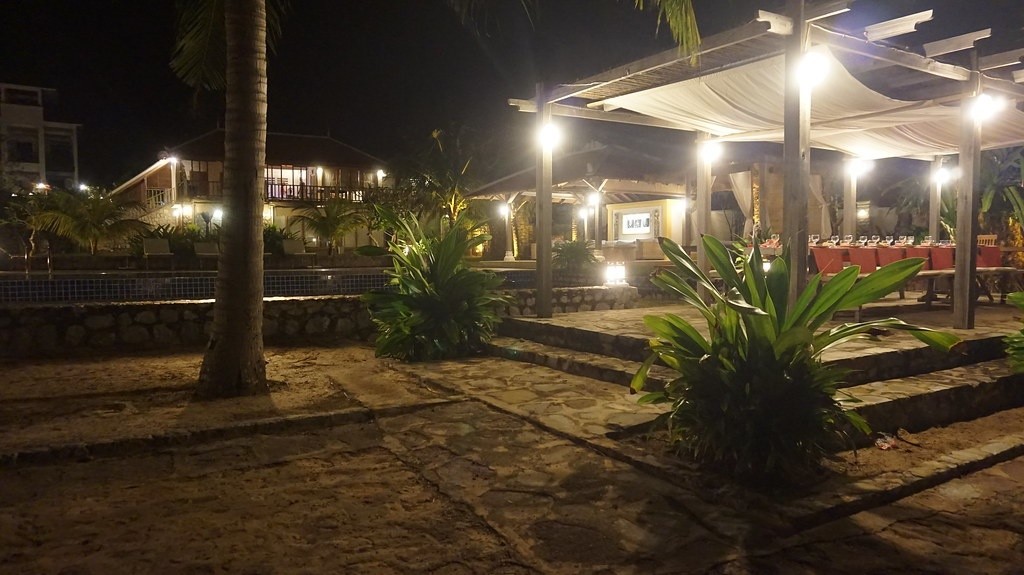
886 236 893 245
771 233 779 247
831 236 839 247
872 236 880 245
860 236 867 246
845 234 853 245
811 235 819 244
899 236 932 247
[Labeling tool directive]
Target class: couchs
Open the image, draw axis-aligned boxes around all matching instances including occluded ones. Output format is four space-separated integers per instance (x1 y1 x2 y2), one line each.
603 238 665 260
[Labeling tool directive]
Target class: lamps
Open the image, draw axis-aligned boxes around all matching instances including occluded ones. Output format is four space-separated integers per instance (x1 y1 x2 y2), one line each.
603 261 629 287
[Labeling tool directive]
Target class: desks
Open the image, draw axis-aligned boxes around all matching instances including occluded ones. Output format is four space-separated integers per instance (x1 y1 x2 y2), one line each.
747 247 1024 305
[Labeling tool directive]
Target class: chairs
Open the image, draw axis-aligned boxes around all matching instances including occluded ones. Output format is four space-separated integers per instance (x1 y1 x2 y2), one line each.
941 234 998 303
143 238 175 271
282 240 316 269
194 242 219 269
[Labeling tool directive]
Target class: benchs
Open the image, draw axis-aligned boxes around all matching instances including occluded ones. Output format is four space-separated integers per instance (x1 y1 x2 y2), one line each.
801 265 1018 321
